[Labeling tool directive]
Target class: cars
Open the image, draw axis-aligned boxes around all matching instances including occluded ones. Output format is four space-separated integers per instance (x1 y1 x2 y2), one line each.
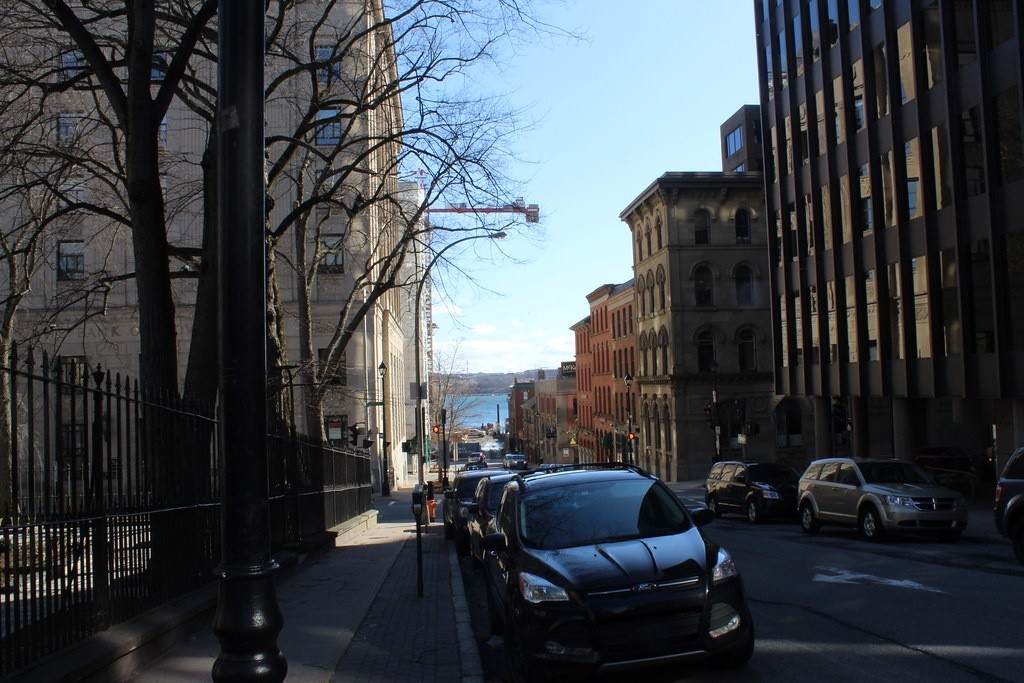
442 469 513 548
539 463 580 474
705 462 803 526
464 452 487 470
466 471 545 575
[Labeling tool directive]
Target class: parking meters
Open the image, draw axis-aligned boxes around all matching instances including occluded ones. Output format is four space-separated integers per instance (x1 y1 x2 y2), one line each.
409 492 426 599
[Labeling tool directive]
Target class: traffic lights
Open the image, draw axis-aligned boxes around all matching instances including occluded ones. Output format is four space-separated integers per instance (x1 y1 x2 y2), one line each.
704 405 717 429
433 427 439 433
629 433 635 439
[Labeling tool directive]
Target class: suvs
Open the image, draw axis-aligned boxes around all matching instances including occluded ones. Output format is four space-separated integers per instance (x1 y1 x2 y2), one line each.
995 446 1024 565
478 461 757 683
797 458 970 543
469 429 486 438
503 454 528 470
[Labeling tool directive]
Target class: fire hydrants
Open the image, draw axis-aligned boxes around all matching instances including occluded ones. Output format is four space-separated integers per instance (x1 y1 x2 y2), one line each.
426 480 437 523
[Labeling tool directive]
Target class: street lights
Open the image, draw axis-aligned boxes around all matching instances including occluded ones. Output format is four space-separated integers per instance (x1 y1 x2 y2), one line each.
414 231 509 523
623 373 633 463
377 361 389 497
707 359 722 462
